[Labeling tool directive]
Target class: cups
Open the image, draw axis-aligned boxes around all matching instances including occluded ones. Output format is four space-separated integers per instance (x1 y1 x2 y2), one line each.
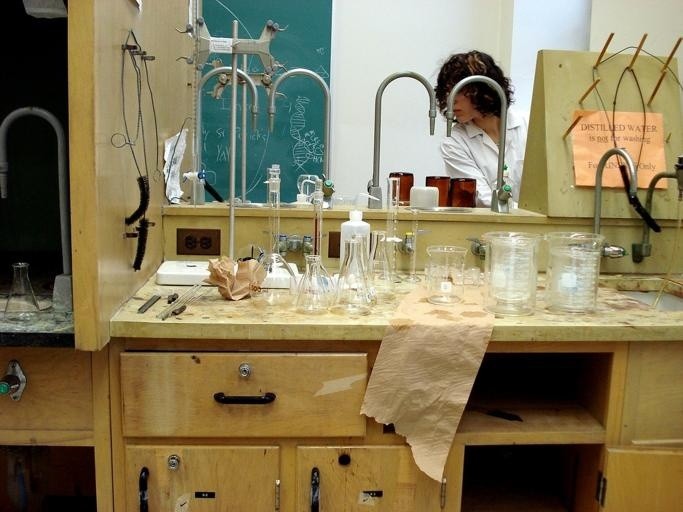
450 178 477 207
480 231 542 318
423 245 469 306
388 172 414 206
425 176 450 207
542 232 605 315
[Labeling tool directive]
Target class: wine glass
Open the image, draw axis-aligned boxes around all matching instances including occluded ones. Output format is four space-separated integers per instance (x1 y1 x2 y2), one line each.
296 173 320 206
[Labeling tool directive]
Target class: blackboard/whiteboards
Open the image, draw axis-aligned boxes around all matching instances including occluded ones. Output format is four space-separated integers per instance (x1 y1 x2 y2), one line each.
197 0 333 207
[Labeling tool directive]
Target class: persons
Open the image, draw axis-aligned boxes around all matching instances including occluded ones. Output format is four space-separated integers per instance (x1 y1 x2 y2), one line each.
431 49 527 207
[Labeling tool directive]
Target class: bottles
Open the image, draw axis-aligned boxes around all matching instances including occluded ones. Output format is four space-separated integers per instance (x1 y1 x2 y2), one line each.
378 176 402 284
350 234 377 309
297 254 330 315
155 281 204 320
310 178 335 303
369 230 397 300
330 239 371 318
4 262 41 326
248 166 298 309
339 210 371 276
404 209 421 283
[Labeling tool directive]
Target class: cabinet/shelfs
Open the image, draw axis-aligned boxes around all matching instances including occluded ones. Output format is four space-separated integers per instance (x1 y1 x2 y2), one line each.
113 347 376 440
117 439 282 509
294 444 447 510
2 349 110 512
456 442 613 510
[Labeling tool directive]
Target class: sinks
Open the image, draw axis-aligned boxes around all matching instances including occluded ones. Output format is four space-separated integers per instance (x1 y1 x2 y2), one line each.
599 279 683 312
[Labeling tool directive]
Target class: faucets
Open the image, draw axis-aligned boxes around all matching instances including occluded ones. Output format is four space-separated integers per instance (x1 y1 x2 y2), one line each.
372 72 436 186
643 156 683 242
195 66 257 171
0 107 70 275
269 68 330 183
594 149 638 234
446 75 508 189
